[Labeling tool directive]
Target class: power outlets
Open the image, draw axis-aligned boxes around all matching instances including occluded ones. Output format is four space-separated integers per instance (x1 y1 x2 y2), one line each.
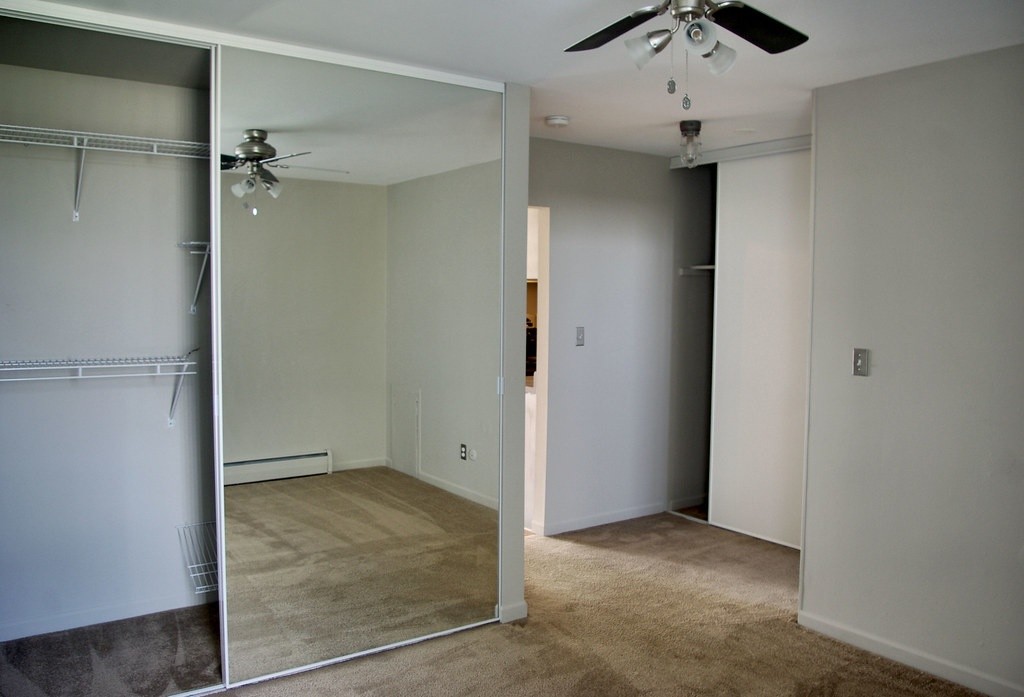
460 443 466 461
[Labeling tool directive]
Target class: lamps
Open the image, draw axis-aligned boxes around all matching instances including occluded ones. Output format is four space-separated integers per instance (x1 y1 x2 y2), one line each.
678 121 704 168
230 165 284 217
624 15 736 110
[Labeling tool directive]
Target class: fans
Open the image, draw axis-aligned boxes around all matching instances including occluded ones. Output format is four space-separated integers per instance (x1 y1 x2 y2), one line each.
220 130 350 182
563 0 810 54
